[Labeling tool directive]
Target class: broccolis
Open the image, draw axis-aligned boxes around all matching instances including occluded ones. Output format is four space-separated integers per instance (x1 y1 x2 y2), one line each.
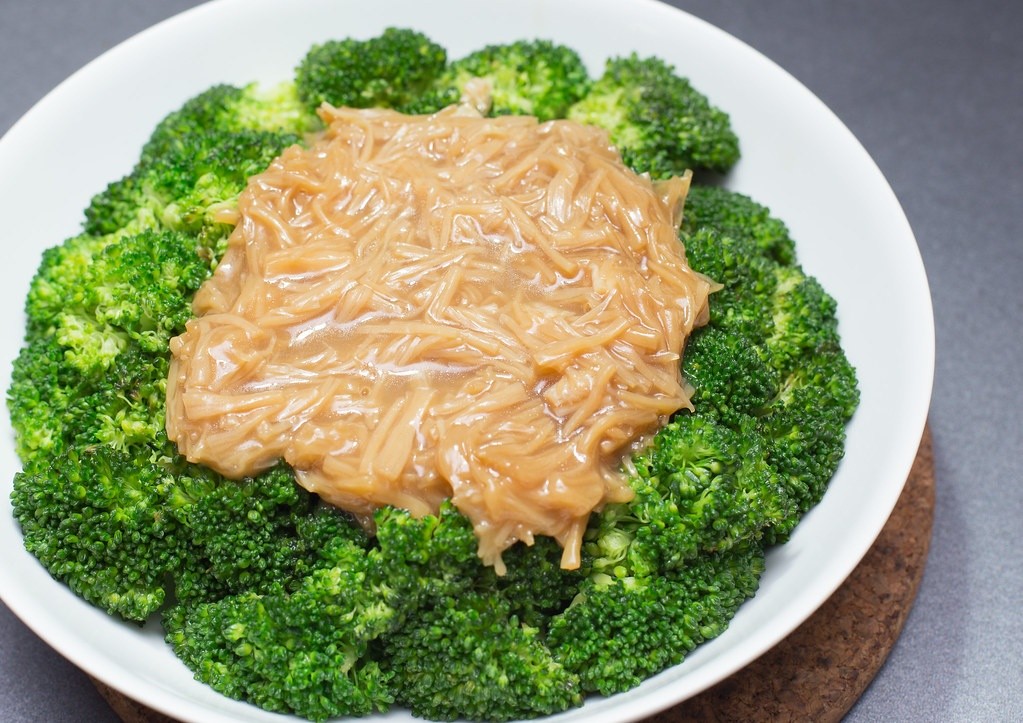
10 29 860 722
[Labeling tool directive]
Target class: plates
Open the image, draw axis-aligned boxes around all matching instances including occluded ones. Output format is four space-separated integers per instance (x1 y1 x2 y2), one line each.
0 0 936 723
